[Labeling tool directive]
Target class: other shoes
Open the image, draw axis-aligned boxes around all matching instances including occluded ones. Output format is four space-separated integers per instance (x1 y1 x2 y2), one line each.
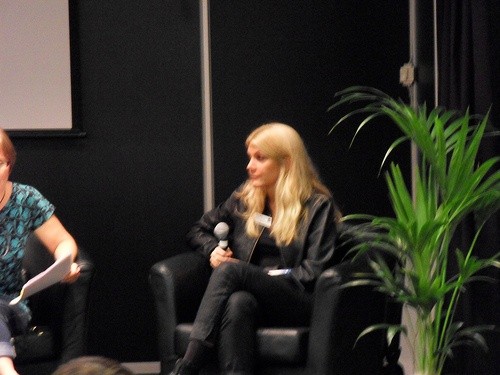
168 359 196 375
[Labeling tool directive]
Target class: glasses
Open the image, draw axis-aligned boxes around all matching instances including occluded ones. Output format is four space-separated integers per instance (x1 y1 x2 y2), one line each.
0 159 9 167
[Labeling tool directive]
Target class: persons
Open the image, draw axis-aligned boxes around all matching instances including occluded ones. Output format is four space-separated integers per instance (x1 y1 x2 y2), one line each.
162 123 346 375
52 355 135 375
0 125 79 375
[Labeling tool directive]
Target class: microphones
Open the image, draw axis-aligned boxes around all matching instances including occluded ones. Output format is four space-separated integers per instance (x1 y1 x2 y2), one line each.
215 221 229 250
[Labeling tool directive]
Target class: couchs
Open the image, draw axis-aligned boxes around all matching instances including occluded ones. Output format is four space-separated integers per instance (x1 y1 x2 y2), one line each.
12 248 95 375
151 224 375 375
